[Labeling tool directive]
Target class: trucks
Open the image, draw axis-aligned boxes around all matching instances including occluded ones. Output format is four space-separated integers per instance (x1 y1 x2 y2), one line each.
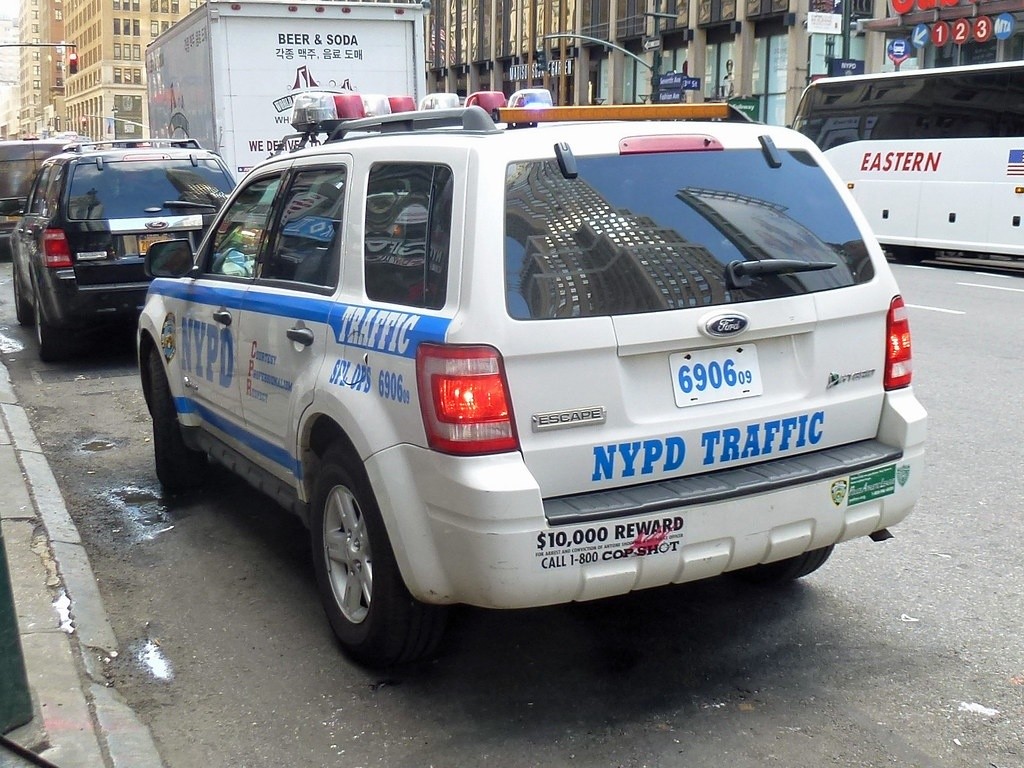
145 0 432 204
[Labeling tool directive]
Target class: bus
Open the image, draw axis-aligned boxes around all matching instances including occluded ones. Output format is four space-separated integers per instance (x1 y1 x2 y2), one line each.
786 61 1024 275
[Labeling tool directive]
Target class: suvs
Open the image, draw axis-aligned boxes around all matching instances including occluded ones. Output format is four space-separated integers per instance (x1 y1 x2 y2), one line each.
0 131 240 362
138 90 929 673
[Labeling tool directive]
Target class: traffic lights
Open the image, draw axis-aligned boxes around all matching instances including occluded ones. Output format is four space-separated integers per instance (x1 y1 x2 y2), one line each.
536 48 549 73
82 120 88 126
69 54 78 76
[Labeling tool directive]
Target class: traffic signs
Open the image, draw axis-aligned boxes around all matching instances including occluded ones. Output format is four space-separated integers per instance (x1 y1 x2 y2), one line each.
641 35 662 52
